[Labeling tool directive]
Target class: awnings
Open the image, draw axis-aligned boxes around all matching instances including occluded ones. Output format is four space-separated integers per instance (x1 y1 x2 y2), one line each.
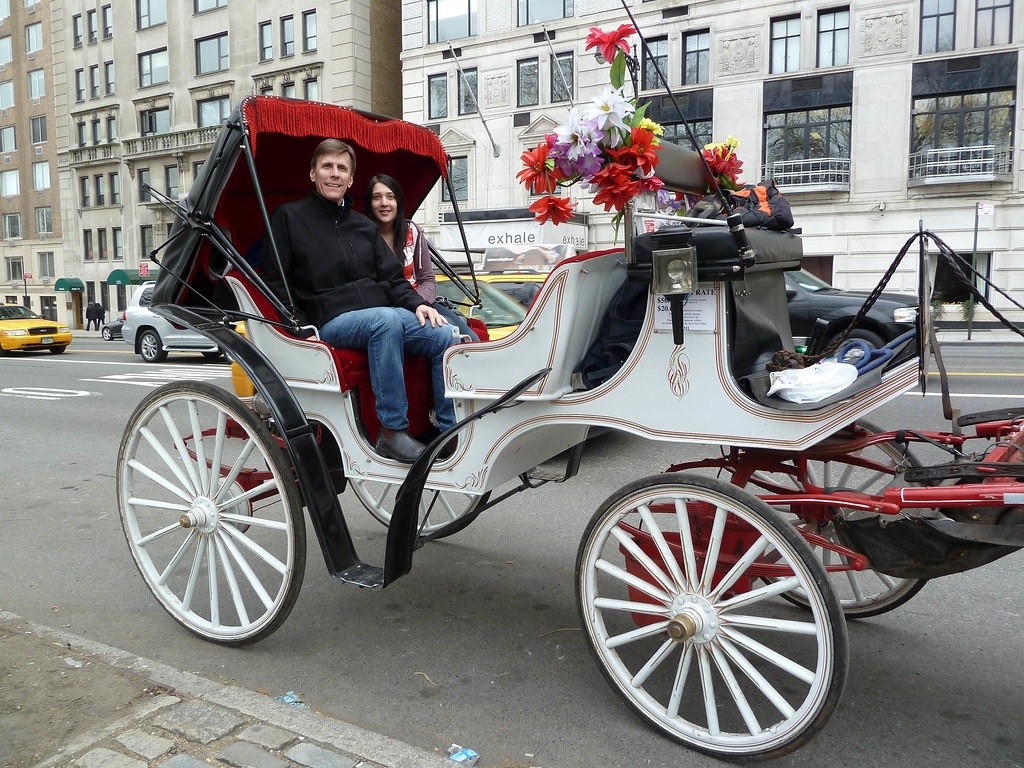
439 209 589 252
55 278 84 291
106 269 159 323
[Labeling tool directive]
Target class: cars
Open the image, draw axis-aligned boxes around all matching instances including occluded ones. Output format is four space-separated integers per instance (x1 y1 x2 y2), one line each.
784 267 939 361
0 301 73 357
101 316 126 341
231 269 551 397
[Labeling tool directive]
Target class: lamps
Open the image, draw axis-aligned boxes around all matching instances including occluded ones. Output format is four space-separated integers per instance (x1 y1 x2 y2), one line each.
595 29 640 106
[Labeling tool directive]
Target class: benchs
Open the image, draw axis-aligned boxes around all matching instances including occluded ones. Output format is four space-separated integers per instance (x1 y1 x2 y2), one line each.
224 216 484 395
620 137 803 281
444 247 626 397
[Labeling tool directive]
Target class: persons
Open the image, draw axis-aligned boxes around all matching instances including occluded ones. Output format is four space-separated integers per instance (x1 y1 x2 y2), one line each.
265 138 460 463
363 173 481 342
85 302 98 331
94 303 105 331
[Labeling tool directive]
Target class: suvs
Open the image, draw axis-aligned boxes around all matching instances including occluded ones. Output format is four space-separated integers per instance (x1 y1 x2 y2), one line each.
121 281 235 366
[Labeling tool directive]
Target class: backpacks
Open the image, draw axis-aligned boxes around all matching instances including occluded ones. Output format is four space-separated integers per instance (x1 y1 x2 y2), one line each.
691 180 794 230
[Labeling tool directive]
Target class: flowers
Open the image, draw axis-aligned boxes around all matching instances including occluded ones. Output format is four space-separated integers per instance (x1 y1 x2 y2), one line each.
522 22 740 228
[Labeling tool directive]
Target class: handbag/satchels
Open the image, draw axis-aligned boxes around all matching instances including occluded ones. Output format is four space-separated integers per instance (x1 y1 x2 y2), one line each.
767 358 857 404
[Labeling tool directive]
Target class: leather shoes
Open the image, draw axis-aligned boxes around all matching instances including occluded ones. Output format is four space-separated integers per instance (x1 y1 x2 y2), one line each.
374 429 425 463
